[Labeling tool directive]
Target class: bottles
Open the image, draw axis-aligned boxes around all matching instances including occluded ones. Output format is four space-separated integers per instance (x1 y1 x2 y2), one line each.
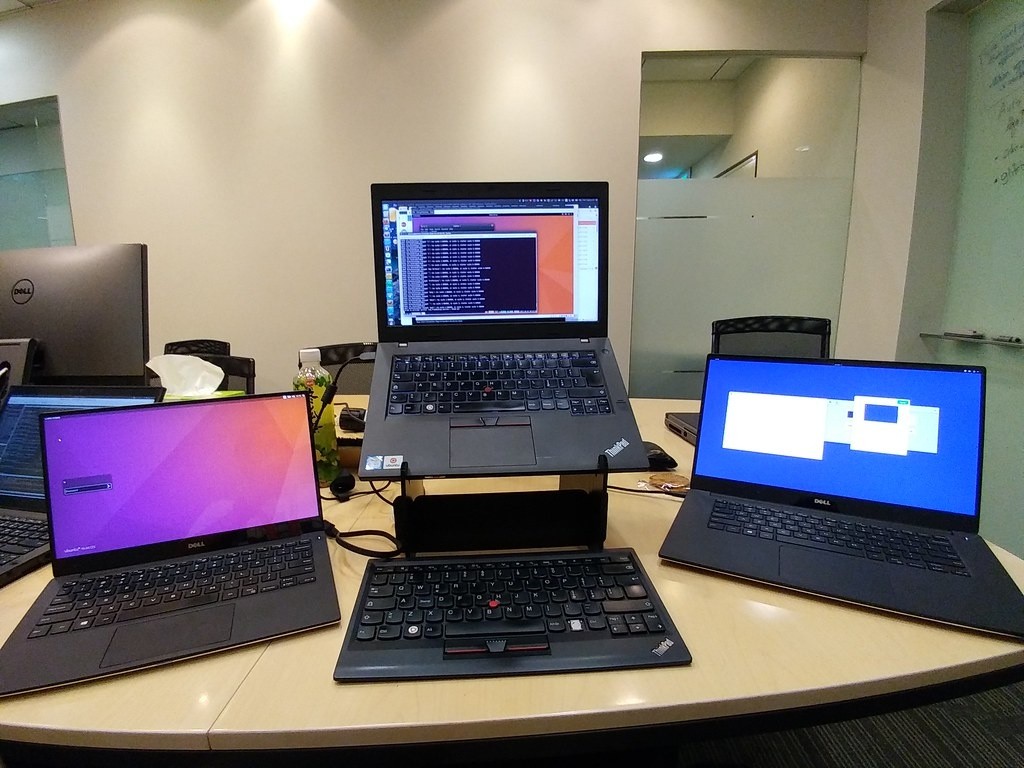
293 347 339 490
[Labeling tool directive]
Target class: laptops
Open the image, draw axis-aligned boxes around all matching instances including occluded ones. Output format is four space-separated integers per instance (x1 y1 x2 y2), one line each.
657 353 1024 640
359 181 651 475
0 391 341 699
0 386 168 589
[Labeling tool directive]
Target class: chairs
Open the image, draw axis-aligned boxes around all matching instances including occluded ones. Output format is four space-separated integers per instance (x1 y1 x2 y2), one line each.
149 353 256 395
297 342 377 395
710 315 831 359
164 339 230 391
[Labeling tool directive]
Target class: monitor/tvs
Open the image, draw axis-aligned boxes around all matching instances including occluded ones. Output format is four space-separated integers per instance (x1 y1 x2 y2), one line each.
0 243 150 393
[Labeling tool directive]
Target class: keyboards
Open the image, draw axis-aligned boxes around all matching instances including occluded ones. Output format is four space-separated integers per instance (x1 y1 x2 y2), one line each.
333 547 692 683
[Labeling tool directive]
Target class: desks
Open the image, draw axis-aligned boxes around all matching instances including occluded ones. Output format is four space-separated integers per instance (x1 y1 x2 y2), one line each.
207 397 1024 768
0 395 382 768
